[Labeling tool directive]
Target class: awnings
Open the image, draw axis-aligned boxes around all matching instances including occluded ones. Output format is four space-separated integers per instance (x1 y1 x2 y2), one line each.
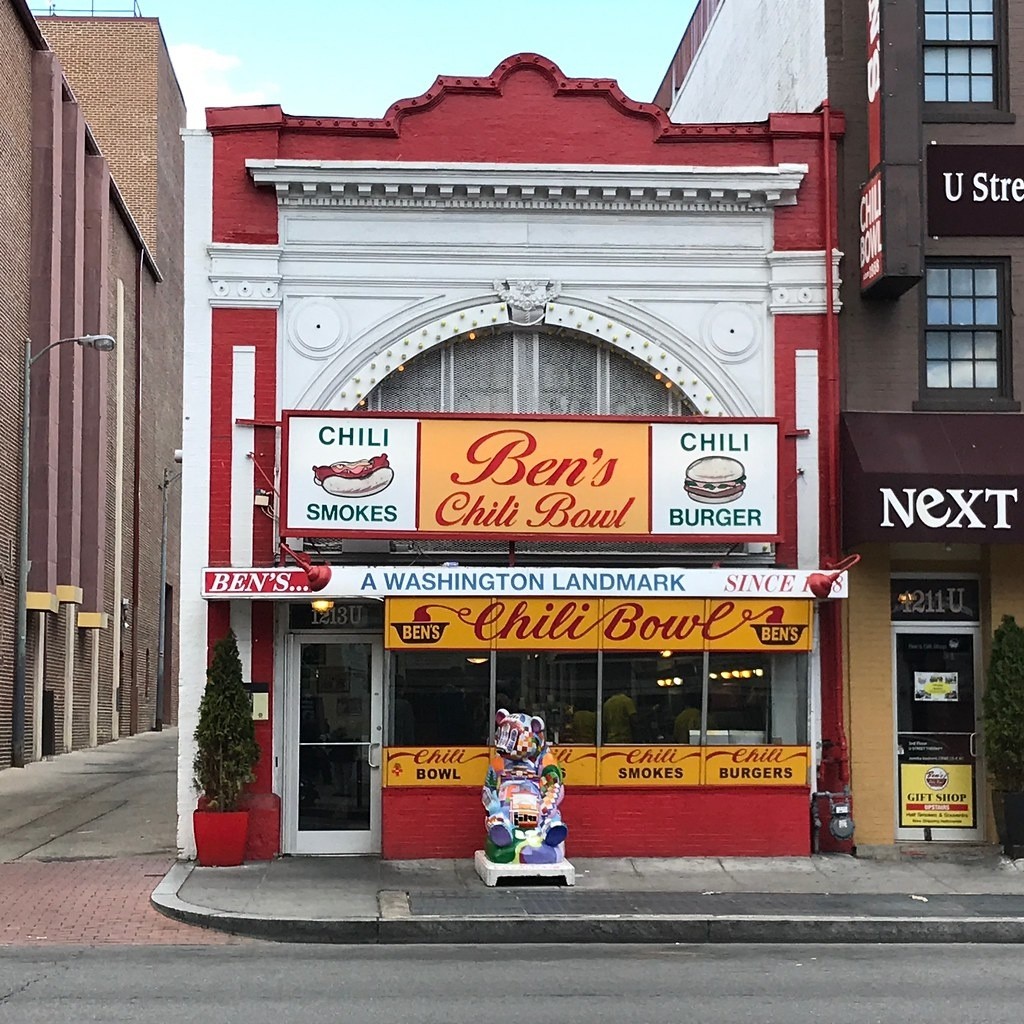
838 410 1024 549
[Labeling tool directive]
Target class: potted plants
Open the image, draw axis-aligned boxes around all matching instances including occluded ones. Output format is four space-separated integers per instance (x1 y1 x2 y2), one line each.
977 613 1024 860
193 627 261 869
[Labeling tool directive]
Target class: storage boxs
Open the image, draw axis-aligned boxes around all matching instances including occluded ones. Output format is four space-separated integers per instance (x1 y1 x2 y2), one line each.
728 730 765 746
689 730 729 746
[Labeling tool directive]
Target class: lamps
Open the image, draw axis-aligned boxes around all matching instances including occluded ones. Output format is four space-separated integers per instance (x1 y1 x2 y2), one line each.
659 650 673 658
281 543 333 593
464 651 489 665
654 673 684 688
808 553 861 600
310 599 334 613
708 664 766 679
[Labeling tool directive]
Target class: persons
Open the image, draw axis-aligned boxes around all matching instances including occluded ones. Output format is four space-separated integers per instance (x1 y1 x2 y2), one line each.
573 685 641 743
674 704 701 743
395 673 415 746
437 667 466 730
